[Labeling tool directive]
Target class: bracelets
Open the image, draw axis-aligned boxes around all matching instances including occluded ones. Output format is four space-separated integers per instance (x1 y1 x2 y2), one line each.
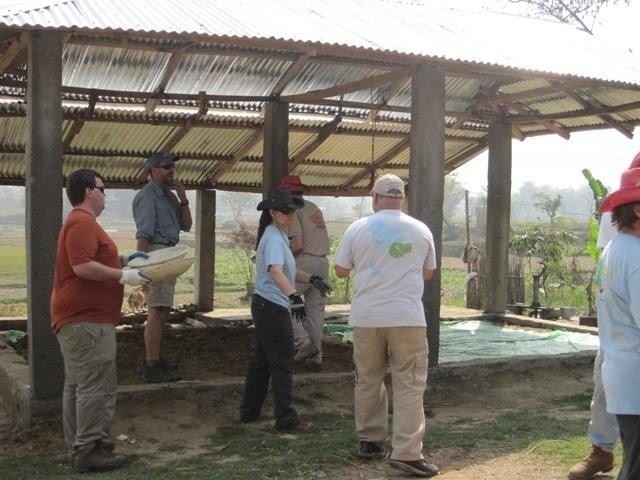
180 198 189 206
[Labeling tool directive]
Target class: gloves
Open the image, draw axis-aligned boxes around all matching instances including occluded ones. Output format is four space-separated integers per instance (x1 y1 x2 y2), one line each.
309 275 333 297
119 269 151 285
288 291 309 323
123 251 149 265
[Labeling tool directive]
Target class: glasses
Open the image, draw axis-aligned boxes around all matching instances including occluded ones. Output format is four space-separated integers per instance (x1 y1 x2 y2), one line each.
95 186 104 193
159 164 173 169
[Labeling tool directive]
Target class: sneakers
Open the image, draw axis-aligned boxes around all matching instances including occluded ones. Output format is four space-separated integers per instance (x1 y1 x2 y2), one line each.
272 421 312 434
391 459 439 476
567 444 614 480
300 359 320 372
71 439 125 473
359 441 385 457
295 343 319 364
144 365 180 382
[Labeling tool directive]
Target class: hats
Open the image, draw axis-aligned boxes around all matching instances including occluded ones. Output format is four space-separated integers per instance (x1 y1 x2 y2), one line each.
257 190 304 210
274 208 295 214
148 151 180 170
597 168 640 212
279 175 312 193
370 173 405 198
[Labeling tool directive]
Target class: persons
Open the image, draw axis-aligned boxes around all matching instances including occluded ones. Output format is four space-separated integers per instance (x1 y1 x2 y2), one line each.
566 151 640 480
240 188 334 432
277 175 331 372
596 167 640 480
131 150 193 382
49 168 152 474
333 172 441 476
